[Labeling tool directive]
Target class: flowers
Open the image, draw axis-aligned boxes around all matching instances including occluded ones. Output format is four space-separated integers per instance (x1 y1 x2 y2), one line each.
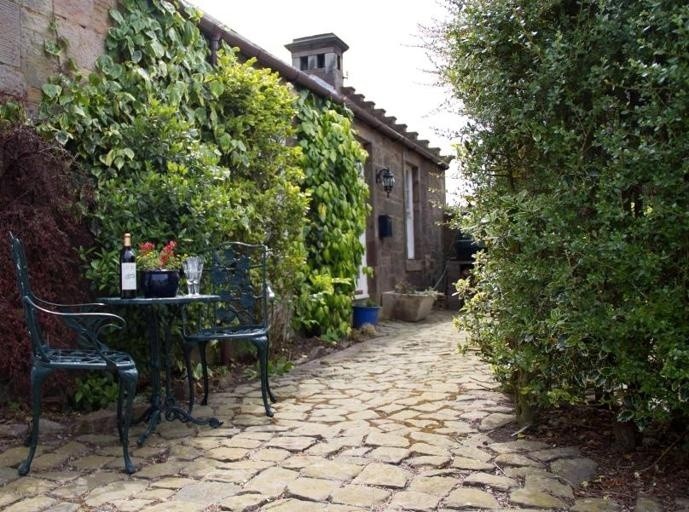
134 239 191 272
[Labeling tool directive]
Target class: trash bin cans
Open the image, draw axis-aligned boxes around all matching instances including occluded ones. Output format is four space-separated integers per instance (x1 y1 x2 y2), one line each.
352 306 381 329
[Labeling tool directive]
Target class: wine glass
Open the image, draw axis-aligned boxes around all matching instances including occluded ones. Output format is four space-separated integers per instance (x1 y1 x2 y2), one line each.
181 256 205 298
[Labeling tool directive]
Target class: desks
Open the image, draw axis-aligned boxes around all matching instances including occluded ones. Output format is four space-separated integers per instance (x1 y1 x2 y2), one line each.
94 294 224 450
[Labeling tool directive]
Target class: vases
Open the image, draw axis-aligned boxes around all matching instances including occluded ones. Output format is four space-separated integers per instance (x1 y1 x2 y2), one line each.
140 269 180 298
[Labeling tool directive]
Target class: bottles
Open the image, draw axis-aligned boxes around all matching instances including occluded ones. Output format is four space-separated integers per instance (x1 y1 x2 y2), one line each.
119 233 137 300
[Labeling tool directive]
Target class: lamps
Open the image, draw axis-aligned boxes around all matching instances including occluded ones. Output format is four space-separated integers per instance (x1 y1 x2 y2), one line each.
375 166 395 199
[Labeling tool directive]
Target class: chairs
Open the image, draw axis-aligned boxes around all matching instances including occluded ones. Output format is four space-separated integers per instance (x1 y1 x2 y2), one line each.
2 230 138 476
178 241 278 422
